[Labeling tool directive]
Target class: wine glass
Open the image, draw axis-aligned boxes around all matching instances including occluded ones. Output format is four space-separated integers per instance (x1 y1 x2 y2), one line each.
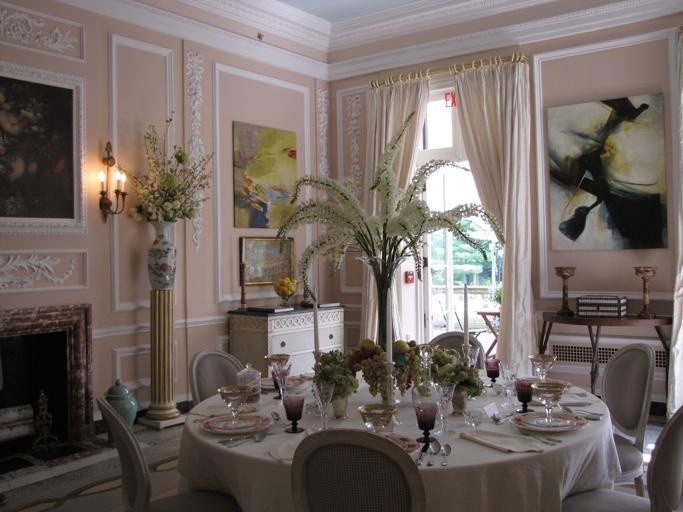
462 346 567 427
357 382 456 442
217 353 336 433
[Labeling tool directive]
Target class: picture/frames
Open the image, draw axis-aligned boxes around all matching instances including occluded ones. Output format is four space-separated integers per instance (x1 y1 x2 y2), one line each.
0 60 89 235
239 236 294 286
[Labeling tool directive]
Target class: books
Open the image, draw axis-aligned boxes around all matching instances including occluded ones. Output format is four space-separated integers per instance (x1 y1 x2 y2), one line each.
247 306 294 313
303 302 341 308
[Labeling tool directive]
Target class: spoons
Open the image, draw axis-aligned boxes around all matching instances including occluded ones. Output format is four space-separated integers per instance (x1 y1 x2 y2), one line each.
227 433 267 448
426 438 451 466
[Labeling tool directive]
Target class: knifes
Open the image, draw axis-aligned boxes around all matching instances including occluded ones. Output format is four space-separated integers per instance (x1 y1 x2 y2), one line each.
417 442 429 466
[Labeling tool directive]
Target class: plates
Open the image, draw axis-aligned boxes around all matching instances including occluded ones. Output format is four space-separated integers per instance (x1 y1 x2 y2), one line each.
386 436 421 456
200 415 272 435
510 411 588 433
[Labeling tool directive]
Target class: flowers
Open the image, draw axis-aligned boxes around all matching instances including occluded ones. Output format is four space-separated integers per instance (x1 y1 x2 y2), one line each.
276 110 506 361
116 108 215 221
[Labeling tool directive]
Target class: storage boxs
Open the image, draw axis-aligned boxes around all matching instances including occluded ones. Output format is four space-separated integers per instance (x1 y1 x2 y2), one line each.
578 295 628 318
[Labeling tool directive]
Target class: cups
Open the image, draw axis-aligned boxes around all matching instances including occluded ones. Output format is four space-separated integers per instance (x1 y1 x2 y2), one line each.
464 411 482 435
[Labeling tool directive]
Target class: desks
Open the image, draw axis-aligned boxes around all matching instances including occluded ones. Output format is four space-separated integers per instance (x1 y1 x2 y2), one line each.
477 312 500 359
539 312 673 401
227 304 346 378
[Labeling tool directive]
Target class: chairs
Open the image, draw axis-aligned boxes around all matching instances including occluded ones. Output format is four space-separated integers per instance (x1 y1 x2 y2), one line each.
190 351 244 406
290 429 427 512
600 343 655 498
95 395 243 511
562 405 683 512
429 332 486 369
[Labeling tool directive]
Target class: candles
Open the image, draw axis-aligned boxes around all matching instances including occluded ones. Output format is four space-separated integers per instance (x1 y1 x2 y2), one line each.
240 263 246 308
100 170 127 192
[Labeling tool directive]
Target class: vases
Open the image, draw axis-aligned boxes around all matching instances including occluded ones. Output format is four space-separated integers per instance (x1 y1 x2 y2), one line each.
355 253 419 361
147 221 178 290
